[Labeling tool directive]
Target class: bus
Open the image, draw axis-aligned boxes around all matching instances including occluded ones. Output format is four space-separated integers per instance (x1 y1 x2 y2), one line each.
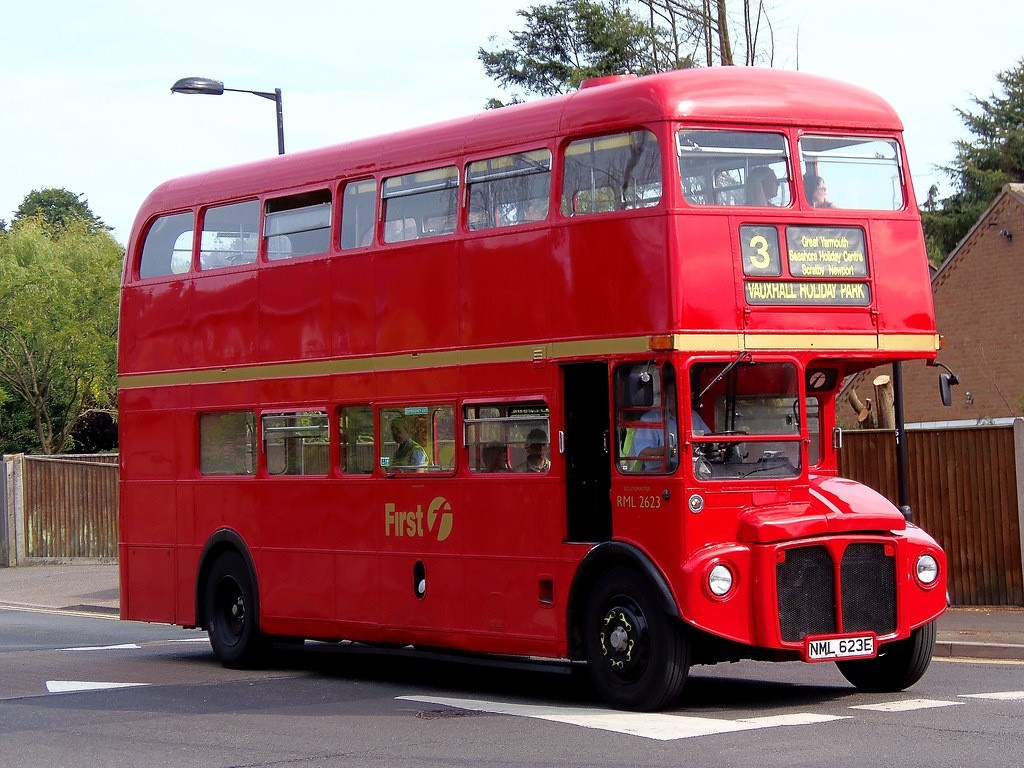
114 67 962 714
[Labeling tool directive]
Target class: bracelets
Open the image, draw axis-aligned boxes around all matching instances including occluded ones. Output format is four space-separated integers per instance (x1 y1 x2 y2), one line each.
827 200 834 206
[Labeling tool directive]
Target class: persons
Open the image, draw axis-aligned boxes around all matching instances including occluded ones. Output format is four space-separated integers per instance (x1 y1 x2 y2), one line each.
742 166 782 208
389 417 431 475
634 383 714 473
480 429 552 475
804 174 840 209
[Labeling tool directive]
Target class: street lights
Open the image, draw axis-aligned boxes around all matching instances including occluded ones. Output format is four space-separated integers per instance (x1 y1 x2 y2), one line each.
169 75 285 151
